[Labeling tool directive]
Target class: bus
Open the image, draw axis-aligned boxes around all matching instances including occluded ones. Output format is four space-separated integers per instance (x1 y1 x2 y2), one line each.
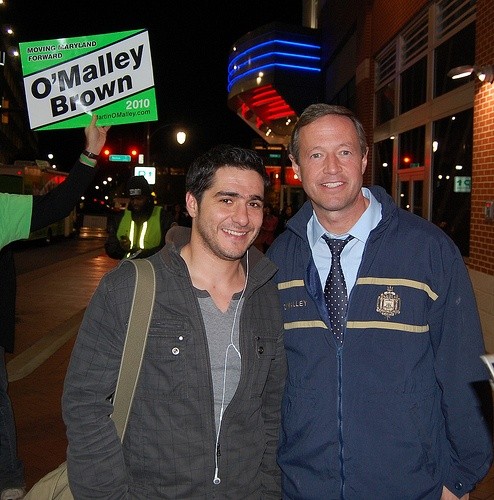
0 164 77 246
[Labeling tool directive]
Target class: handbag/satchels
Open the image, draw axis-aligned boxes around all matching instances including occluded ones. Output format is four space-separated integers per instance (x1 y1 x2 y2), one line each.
23 461 74 500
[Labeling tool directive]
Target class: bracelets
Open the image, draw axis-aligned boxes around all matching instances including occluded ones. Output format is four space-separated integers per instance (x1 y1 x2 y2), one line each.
82 149 100 160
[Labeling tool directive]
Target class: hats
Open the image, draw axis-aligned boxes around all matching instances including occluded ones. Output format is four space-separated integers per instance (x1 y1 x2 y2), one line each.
125 176 151 196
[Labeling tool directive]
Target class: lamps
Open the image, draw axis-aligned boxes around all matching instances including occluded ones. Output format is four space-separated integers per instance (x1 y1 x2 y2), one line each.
447 63 493 83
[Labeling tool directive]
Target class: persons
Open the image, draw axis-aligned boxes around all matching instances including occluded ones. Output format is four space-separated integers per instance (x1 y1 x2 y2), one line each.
264 104 494 500
252 204 295 254
104 176 192 260
58 146 289 500
0 114 111 500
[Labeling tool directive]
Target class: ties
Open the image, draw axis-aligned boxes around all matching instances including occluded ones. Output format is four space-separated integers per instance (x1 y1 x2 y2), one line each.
322 234 355 346
